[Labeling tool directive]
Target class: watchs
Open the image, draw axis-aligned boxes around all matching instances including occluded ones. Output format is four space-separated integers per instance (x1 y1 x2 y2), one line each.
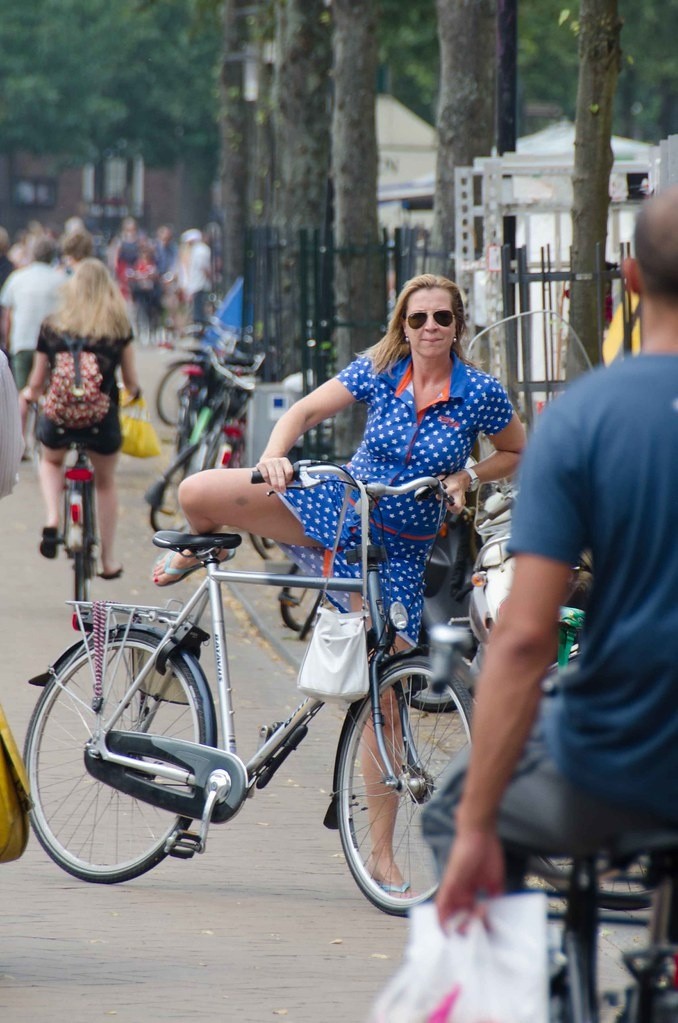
462 467 480 493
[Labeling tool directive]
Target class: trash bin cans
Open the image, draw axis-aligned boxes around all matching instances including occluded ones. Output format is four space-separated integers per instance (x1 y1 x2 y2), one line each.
246 384 298 468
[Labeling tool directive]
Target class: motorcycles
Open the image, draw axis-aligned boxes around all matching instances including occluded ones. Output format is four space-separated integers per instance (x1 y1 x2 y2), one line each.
469 307 598 645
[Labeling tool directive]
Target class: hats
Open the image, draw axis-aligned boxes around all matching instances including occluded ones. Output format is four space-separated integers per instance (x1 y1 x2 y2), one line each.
181 228 202 242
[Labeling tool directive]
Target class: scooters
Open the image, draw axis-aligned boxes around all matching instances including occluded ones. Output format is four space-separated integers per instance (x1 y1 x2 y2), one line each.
400 504 477 712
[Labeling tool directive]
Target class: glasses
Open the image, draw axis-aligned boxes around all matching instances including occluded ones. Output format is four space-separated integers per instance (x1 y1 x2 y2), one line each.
406 310 456 330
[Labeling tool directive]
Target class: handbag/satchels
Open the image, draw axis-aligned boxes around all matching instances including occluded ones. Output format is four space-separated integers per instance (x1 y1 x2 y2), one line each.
373 890 547 1023
298 607 371 705
118 392 160 458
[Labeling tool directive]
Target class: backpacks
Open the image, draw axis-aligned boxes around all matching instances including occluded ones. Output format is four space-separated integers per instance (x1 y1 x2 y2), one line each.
0 705 35 864
42 330 113 429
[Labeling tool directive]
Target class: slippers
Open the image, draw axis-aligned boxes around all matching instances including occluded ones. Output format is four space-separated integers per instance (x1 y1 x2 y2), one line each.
40 526 58 561
379 880 415 904
153 545 236 587
100 564 122 579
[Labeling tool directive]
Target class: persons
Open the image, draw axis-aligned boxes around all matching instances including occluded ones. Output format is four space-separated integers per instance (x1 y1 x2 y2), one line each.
422 187 678 1022
151 275 526 899
0 219 217 581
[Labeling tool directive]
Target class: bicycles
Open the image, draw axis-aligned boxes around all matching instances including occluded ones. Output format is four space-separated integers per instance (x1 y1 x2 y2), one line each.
488 488 678 1023
149 315 323 632
28 391 141 607
23 459 475 916
